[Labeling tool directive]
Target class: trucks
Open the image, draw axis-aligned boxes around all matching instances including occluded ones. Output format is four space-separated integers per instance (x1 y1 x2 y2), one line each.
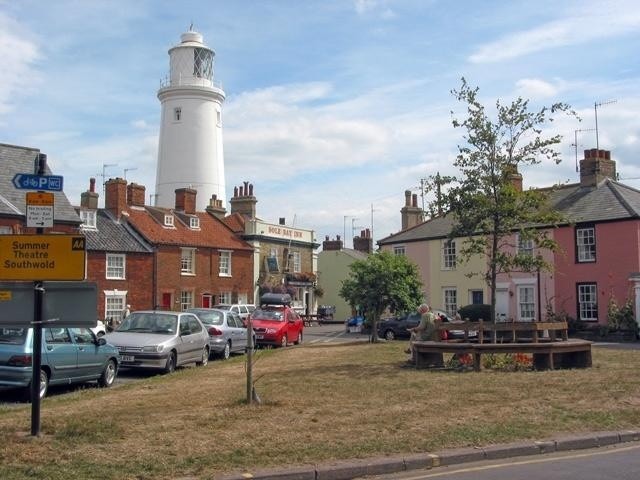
318 303 336 325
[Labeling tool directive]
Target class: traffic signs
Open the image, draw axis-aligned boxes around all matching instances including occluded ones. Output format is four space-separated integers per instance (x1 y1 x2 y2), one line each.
11 174 63 192
25 205 54 230
26 192 55 205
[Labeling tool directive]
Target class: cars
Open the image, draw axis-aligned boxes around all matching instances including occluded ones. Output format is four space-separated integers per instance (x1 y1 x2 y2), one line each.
89 292 304 375
345 308 374 334
0 321 120 403
375 308 479 343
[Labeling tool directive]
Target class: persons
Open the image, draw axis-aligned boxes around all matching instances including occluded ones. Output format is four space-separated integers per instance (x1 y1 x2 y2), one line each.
122 304 131 321
428 306 447 340
404 303 435 354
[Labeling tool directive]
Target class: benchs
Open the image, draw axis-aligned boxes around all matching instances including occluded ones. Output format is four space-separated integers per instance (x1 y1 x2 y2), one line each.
411 321 595 372
299 314 323 327
